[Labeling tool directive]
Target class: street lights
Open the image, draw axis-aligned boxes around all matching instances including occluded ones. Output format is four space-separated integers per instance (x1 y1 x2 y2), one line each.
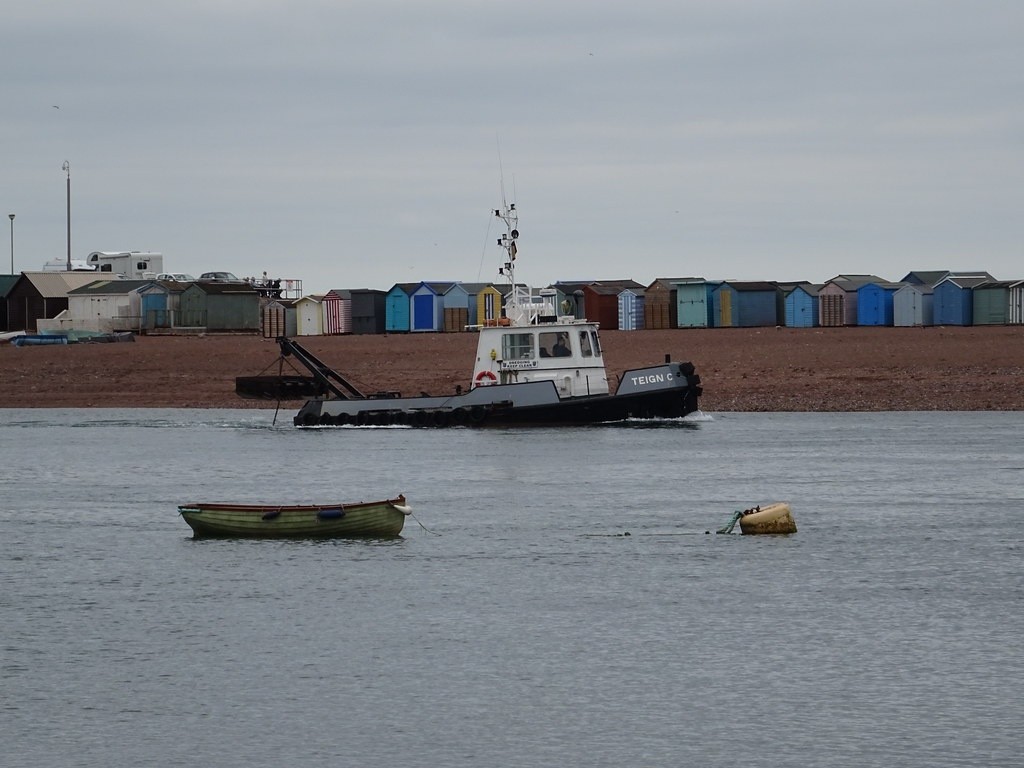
8 209 15 275
62 158 71 271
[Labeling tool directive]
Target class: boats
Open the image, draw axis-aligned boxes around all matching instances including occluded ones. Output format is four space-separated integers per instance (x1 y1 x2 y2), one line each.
293 130 705 426
177 493 413 538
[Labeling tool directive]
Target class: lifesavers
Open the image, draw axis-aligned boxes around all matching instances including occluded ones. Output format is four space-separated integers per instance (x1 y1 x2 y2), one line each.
336 412 351 425
320 413 331 425
375 412 390 426
357 412 370 424
469 406 486 422
450 407 467 425
394 412 409 425
431 411 447 428
302 412 318 425
476 371 497 387
412 411 429 427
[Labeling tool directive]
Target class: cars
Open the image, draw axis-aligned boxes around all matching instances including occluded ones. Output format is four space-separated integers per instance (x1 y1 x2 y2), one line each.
199 271 238 281
155 273 196 282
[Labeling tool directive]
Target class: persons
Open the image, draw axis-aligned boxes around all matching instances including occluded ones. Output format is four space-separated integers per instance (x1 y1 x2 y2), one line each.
553 336 570 357
245 271 281 288
